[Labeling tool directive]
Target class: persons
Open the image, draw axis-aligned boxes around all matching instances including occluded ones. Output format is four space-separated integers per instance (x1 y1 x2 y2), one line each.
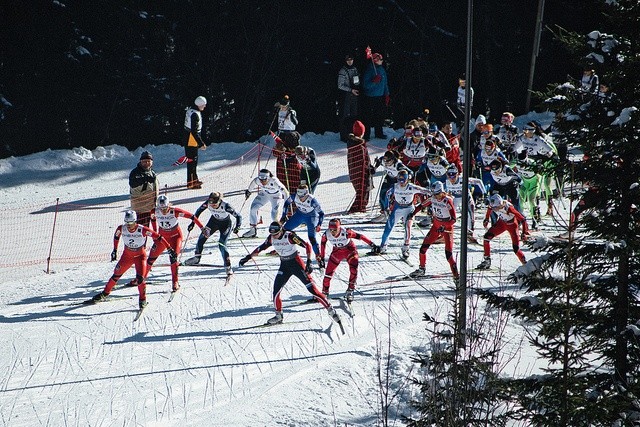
360 53 388 142
130 195 207 290
240 221 341 321
295 146 320 196
184 95 207 190
242 169 292 238
187 191 242 275
456 76 474 120
371 113 599 285
347 120 375 214
240 184 324 266
129 151 158 243
318 218 380 304
93 210 177 308
275 94 299 136
337 57 360 143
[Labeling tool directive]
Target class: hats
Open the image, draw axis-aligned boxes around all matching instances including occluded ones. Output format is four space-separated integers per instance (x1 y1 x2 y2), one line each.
329 219 340 230
139 151 153 161
297 188 308 198
475 114 487 125
490 193 503 207
157 195 170 208
397 173 409 181
480 124 494 132
269 225 280 234
353 120 365 136
279 95 290 106
413 130 424 138
431 181 445 195
429 125 437 132
194 96 207 105
345 53 354 60
484 143 494 152
501 115 512 123
124 210 138 222
447 168 458 179
258 172 270 181
372 53 383 63
405 129 414 136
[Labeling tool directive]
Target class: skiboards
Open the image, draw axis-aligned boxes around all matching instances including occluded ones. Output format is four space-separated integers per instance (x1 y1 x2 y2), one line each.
366 245 414 267
267 250 324 275
48 292 148 320
368 213 389 225
402 269 459 291
264 308 345 336
303 292 356 318
465 231 484 249
180 257 233 287
120 278 180 302
477 257 529 278
228 227 280 242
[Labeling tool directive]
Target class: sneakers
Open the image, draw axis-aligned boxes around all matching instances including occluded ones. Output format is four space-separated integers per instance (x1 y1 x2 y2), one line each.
187 181 202 189
419 217 433 225
375 133 388 140
93 291 110 301
270 249 279 255
327 305 341 322
371 210 389 222
185 254 201 265
410 266 425 277
312 292 329 300
224 266 234 275
193 180 203 185
346 288 354 302
477 256 491 268
401 244 411 258
559 231 574 239
131 277 147 286
374 243 387 256
240 254 251 266
316 253 322 262
433 234 446 243
172 280 180 291
243 230 257 238
138 299 146 308
267 311 283 324
467 232 477 243
